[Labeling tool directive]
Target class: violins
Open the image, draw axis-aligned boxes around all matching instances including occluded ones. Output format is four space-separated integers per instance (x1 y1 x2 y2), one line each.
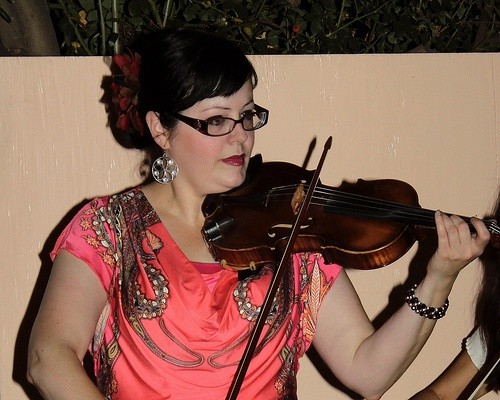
201 161 500 267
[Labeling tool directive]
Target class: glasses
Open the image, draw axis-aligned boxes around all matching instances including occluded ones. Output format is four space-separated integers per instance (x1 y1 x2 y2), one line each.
160 104 269 137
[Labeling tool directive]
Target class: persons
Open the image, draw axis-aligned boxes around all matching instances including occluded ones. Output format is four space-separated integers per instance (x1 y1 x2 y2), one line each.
26 24 490 400
407 192 500 400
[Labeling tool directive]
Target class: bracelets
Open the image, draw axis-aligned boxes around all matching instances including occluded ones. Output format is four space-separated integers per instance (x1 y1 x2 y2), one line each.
404 283 449 320
